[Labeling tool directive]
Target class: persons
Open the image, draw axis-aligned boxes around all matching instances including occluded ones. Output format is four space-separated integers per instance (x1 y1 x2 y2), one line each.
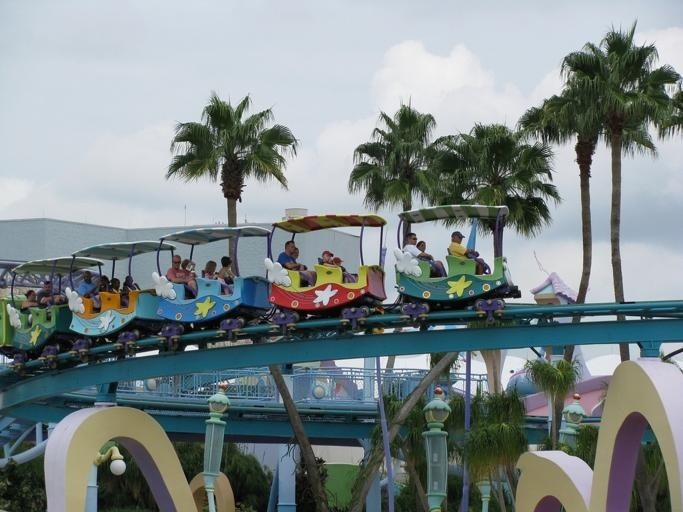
167 255 197 293
77 271 103 309
416 240 426 251
123 275 143 296
277 241 313 288
110 277 123 295
37 281 66 308
181 259 196 278
288 248 316 286
318 251 349 284
97 276 111 292
205 261 232 296
20 290 42 313
219 256 238 284
331 256 355 283
403 233 447 280
448 231 485 275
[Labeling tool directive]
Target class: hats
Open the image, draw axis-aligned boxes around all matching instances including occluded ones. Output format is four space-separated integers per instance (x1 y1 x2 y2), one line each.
333 257 344 263
323 251 334 257
452 231 465 238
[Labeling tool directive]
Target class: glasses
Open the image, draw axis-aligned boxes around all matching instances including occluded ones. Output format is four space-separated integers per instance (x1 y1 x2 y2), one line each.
411 238 418 240
173 261 182 264
